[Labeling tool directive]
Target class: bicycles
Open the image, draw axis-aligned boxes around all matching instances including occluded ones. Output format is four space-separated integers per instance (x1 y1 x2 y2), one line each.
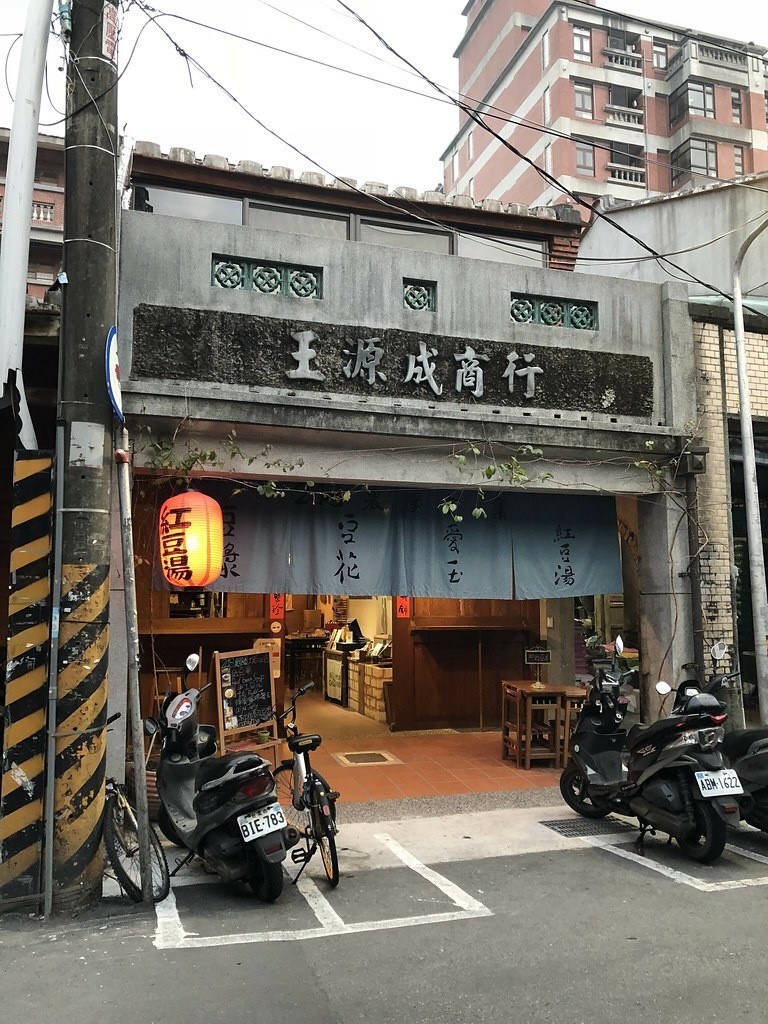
106 713 171 904
257 681 342 889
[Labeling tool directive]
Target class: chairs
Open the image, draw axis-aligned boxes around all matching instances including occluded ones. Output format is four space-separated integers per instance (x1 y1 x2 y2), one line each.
502 683 555 769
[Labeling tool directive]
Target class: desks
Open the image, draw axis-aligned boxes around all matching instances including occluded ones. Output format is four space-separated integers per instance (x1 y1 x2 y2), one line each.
284 634 330 690
502 680 566 771
561 685 587 768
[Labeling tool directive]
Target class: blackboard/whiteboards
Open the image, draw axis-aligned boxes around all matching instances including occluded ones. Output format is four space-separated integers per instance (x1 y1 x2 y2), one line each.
211 646 276 737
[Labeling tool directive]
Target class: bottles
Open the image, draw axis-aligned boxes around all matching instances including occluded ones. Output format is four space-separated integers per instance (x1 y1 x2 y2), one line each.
190 603 197 618
198 594 207 618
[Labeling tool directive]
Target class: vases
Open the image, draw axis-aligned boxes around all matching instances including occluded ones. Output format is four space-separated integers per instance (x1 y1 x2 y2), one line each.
127 767 161 823
258 731 270 743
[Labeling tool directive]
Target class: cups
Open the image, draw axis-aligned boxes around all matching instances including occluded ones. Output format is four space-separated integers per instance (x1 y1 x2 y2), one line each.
258 730 269 743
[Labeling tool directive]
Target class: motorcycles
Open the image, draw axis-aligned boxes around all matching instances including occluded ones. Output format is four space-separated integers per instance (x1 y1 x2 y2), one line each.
140 653 301 904
655 640 768 836
560 635 745 864
